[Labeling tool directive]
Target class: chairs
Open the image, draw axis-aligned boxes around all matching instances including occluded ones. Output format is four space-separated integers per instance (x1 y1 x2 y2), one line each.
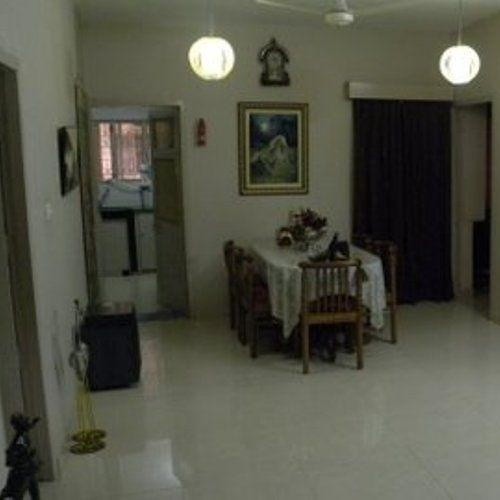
223 234 398 373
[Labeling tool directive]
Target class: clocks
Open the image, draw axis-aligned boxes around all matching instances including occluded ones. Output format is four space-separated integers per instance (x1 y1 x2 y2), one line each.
256 35 293 87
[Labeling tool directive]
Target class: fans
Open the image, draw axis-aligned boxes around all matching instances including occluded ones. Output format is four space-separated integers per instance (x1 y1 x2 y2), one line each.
249 1 424 29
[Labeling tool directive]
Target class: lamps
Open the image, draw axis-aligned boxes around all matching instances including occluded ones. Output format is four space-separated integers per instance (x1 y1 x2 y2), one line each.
186 1 237 81
438 0 481 85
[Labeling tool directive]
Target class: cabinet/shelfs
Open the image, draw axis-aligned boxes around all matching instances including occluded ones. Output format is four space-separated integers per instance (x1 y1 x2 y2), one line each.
83 298 142 392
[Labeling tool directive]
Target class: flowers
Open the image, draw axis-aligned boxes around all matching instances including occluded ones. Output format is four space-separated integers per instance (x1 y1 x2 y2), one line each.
280 206 331 242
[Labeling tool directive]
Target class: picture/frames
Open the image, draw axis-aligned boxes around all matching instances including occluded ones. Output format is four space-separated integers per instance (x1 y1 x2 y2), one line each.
58 126 82 197
236 100 310 195
